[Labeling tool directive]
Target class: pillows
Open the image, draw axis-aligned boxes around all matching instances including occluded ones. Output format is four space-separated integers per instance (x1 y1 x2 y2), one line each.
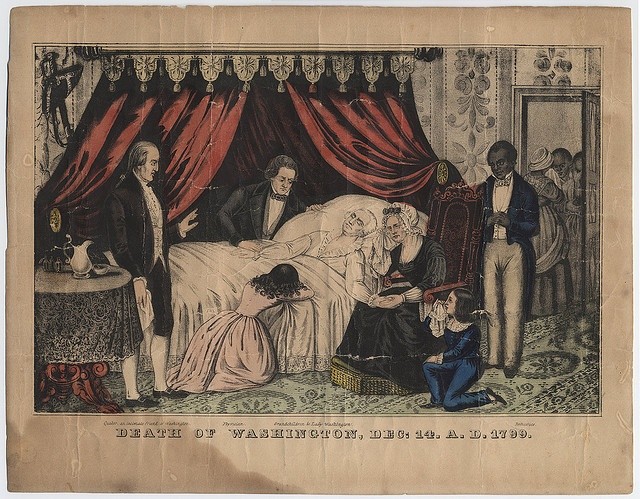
272 192 426 263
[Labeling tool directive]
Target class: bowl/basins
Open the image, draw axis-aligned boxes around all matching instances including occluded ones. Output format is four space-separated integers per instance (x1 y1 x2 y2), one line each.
92 262 110 275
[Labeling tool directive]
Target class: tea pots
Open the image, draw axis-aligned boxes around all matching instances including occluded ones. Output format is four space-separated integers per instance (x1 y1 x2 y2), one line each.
62 233 94 280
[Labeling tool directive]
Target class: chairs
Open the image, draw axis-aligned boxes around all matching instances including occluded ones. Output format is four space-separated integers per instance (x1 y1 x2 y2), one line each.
378 179 484 313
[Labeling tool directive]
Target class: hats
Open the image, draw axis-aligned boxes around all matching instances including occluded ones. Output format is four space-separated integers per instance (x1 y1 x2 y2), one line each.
382 203 423 237
529 148 553 172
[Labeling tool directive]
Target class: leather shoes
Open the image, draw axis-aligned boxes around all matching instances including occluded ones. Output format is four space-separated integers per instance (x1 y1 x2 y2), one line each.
486 362 502 370
504 364 517 378
125 396 156 407
153 388 190 399
484 387 508 406
421 402 443 409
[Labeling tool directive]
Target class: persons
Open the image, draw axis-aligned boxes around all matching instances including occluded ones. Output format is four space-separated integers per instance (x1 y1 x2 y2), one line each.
218 155 324 253
336 202 447 393
166 263 315 394
545 148 575 319
476 140 540 379
521 146 573 317
105 141 199 408
252 208 390 309
562 152 585 320
421 288 507 412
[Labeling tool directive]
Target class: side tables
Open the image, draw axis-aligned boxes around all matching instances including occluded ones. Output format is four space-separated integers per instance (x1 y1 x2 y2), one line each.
34 260 144 414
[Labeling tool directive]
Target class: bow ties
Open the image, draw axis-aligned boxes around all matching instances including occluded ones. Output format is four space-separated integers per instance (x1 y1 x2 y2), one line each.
271 192 288 203
145 181 155 188
493 179 510 187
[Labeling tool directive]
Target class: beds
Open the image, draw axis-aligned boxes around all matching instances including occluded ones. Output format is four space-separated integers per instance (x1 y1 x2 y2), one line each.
102 159 465 378
